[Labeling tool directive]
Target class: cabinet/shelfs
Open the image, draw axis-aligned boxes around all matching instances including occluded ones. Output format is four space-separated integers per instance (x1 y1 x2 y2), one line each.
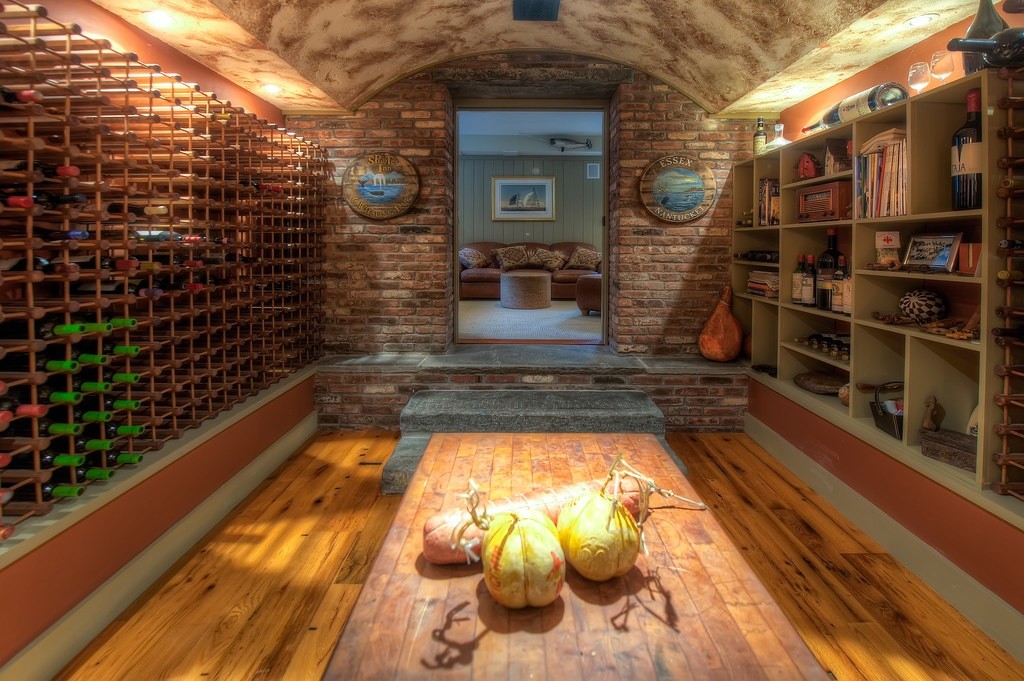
729 64 1024 503
0 0 328 541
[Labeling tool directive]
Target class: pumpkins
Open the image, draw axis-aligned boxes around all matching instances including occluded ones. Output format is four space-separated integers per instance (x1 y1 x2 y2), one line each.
480 490 640 610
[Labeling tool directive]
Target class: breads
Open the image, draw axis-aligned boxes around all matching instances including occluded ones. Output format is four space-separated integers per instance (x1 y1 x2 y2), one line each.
422 475 649 565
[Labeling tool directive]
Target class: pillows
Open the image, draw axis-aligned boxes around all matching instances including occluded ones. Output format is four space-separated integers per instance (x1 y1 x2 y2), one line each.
459 244 602 271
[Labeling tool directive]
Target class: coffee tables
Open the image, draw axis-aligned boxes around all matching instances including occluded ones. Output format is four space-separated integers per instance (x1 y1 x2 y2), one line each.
500 273 551 309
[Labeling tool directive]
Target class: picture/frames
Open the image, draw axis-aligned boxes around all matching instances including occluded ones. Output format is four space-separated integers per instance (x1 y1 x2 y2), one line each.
902 232 962 271
491 175 556 221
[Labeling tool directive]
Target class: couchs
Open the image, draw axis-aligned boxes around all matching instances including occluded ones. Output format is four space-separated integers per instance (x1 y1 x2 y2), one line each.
458 242 602 300
576 274 601 315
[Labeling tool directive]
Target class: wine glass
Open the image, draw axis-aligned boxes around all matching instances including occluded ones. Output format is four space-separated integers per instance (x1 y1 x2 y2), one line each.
929 50 954 85
908 62 931 94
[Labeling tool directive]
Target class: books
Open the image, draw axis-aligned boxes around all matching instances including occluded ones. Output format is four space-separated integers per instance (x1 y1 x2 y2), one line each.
758 178 780 227
745 270 780 301
855 127 908 220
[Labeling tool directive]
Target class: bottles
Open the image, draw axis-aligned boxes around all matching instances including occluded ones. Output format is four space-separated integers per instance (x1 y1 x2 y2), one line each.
752 117 767 156
81 225 165 244
792 332 850 362
791 228 852 316
137 229 231 245
0 318 144 501
0 256 80 275
946 0 1024 75
217 179 283 192
734 250 779 262
74 202 169 217
736 208 753 226
950 90 982 211
0 85 45 103
0 223 89 242
0 160 81 179
801 81 910 137
990 92 1024 335
49 251 263 300
7 191 86 210
757 124 792 154
0 190 34 209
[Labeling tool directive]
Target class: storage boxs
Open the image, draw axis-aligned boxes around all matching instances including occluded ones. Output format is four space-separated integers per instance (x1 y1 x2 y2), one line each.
959 243 982 274
920 428 977 472
